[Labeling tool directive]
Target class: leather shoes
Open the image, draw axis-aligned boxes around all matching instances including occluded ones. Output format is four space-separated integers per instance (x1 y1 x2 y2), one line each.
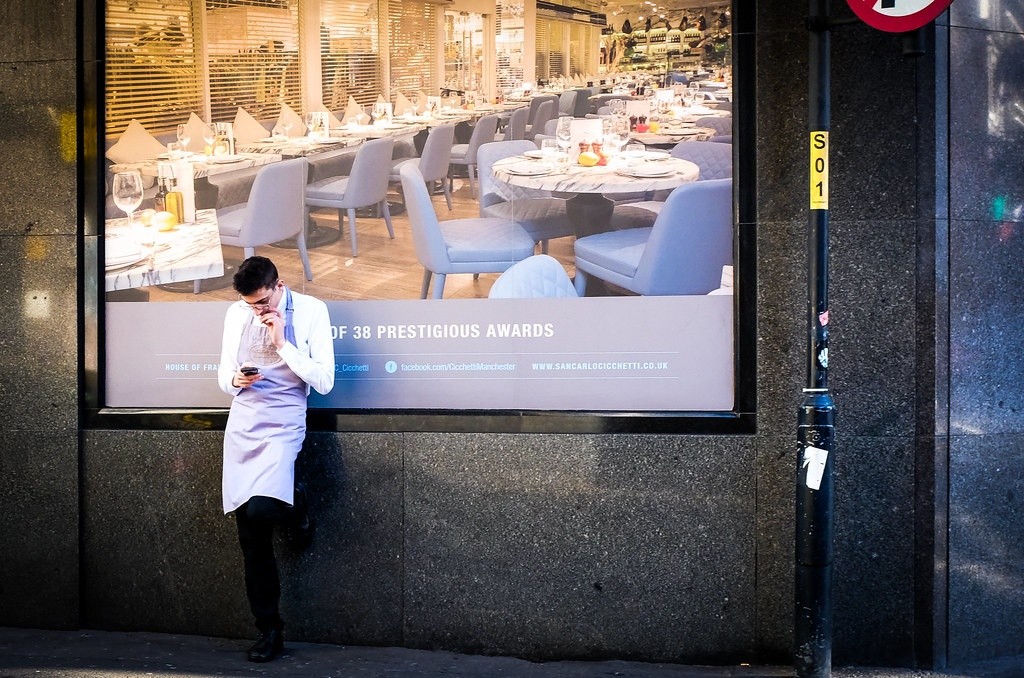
248 630 284 663
285 483 312 552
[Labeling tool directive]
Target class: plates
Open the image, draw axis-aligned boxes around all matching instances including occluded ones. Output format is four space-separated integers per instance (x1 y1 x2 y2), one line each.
616 166 677 179
261 137 288 143
204 155 245 164
157 151 193 158
503 167 556 177
313 137 342 144
105 238 149 272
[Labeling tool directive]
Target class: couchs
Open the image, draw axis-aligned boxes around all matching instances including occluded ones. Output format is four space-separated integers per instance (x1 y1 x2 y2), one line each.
106 89 455 218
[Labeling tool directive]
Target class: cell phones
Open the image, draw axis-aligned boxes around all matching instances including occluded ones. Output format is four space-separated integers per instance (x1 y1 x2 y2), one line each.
240 367 258 375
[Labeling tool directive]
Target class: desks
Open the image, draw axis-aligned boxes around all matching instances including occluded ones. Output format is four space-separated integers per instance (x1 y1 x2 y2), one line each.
492 149 702 297
544 67 649 94
442 98 510 180
392 109 477 196
510 92 556 104
679 107 731 123
328 111 423 220
106 206 227 294
105 153 289 301
628 121 716 149
703 99 726 109
488 97 532 113
235 132 367 250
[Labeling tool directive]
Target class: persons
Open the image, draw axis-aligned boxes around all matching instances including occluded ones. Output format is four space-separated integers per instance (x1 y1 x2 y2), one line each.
218 256 335 664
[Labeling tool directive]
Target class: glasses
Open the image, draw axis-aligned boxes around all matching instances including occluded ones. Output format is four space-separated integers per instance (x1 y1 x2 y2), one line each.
238 289 276 312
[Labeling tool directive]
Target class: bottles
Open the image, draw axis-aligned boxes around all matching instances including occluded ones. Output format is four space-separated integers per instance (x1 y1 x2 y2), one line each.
154 176 185 224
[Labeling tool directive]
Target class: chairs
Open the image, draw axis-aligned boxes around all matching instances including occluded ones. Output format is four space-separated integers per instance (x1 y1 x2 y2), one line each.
194 87 731 298
611 140 733 231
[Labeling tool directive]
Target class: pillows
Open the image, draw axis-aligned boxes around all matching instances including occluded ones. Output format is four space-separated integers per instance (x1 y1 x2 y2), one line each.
105 116 168 190
271 99 309 139
339 98 372 126
231 106 271 147
370 95 393 119
415 87 434 115
173 110 221 155
395 90 417 117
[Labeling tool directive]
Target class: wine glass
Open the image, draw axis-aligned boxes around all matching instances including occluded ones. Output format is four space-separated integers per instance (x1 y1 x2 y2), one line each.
411 96 420 117
353 104 365 126
500 59 733 167
112 172 144 227
305 112 317 132
372 103 385 122
176 124 192 160
202 122 219 158
282 116 292 138
449 92 457 110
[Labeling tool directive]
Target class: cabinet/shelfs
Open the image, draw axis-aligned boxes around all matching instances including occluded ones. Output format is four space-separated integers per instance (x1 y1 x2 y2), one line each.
630 30 648 59
683 25 705 58
648 30 667 58
667 27 683 59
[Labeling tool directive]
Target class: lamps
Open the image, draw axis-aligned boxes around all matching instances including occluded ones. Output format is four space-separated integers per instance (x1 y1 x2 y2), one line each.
638 0 668 23
613 5 625 17
711 5 732 16
